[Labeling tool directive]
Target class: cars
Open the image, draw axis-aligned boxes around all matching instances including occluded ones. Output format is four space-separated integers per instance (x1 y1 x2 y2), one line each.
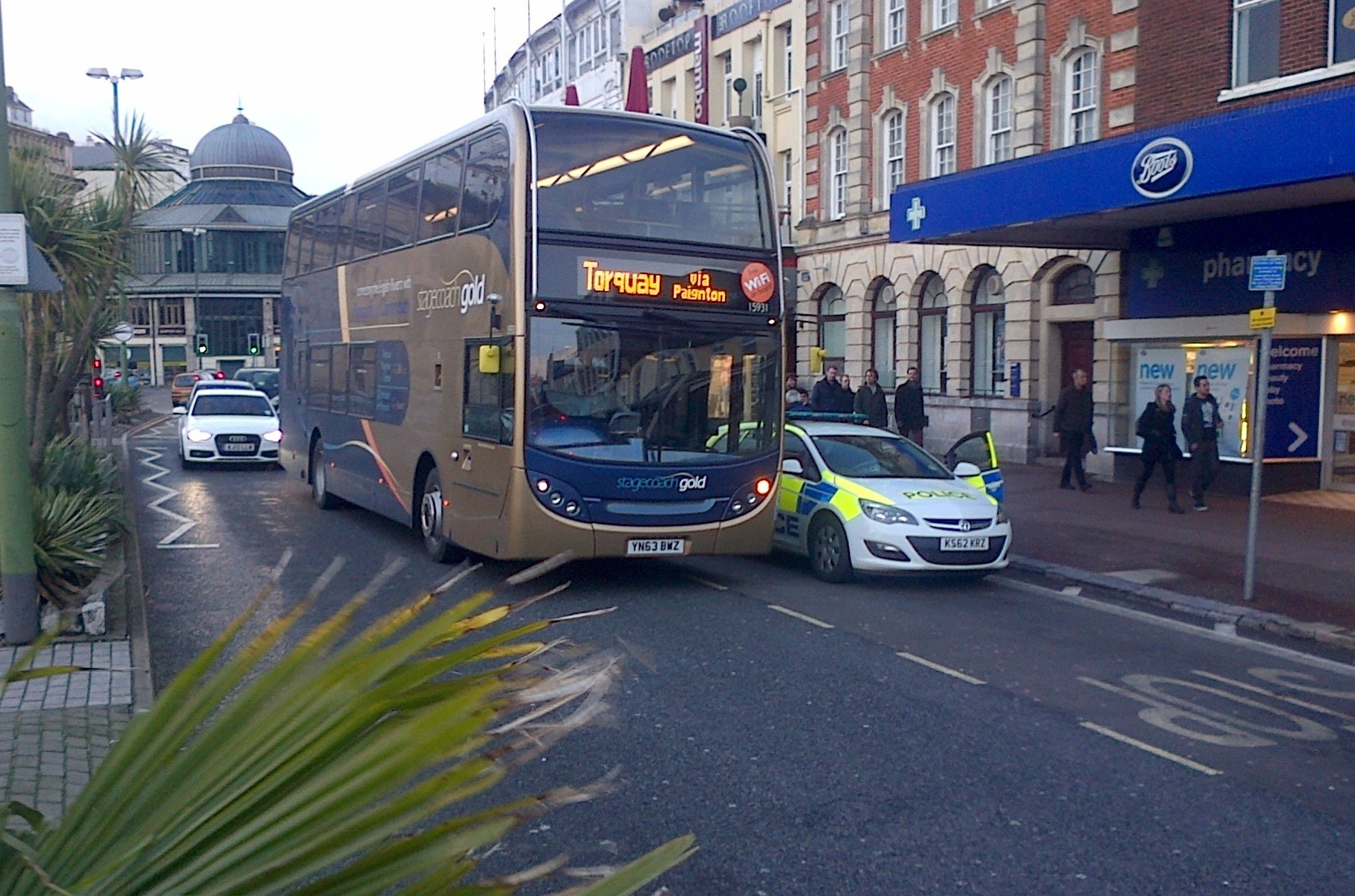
232 369 279 412
172 390 282 469
186 379 256 411
710 411 1014 583
104 370 141 392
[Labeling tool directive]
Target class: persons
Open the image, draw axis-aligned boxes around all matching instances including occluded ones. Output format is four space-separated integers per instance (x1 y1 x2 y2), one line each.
1181 375 1224 513
127 374 141 411
520 354 571 424
750 365 924 448
1052 369 1093 491
1132 383 1184 514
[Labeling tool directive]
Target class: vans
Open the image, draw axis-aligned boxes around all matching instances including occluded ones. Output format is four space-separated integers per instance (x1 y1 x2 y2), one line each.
170 374 211 407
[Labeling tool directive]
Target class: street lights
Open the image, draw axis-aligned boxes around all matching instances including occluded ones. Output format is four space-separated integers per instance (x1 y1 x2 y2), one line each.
181 227 210 369
85 65 148 205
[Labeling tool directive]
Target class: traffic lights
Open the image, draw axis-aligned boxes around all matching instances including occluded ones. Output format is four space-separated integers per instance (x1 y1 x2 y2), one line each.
196 334 210 356
94 360 101 369
94 378 103 398
248 334 260 357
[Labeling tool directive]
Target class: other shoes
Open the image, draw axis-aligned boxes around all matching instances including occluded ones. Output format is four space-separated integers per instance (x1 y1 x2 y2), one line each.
1079 483 1092 491
1168 504 1185 514
1130 495 1140 509
1193 501 1208 513
1059 483 1075 491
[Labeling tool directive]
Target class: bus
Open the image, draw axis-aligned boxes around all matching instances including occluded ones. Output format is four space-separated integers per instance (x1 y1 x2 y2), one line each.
279 97 829 567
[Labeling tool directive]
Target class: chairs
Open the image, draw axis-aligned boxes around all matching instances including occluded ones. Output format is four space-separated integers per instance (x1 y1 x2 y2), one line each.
831 448 901 472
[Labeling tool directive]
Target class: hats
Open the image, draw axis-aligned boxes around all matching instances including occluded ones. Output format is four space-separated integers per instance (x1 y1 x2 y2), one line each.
786 372 799 379
